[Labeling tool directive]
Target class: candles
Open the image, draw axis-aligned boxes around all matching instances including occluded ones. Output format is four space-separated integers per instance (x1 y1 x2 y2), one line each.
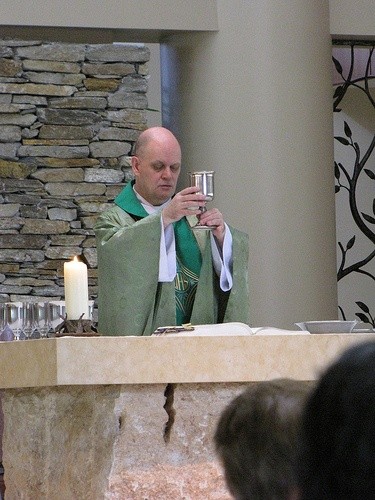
63 255 90 320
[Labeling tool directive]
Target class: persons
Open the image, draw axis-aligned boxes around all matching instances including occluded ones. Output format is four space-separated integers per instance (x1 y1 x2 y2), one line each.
216 340 375 500
93 126 249 336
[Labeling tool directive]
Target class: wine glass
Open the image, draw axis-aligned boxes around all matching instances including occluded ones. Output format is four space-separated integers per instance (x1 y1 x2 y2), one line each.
188 171 217 229
0 300 95 342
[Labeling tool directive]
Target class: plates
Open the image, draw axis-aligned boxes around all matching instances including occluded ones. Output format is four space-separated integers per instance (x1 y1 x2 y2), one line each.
291 321 359 333
